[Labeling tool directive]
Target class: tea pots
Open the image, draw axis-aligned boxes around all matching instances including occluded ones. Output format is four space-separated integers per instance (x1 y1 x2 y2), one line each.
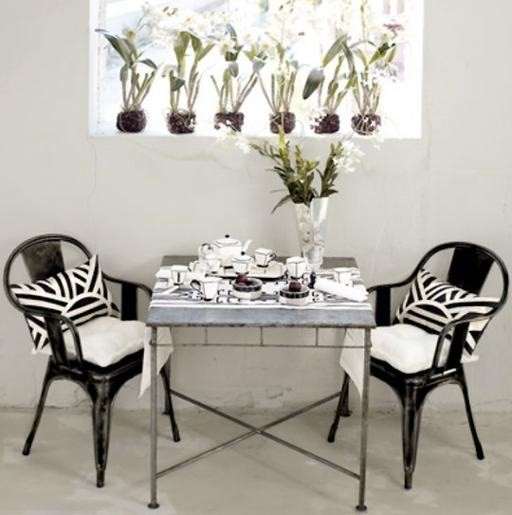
198 235 252 269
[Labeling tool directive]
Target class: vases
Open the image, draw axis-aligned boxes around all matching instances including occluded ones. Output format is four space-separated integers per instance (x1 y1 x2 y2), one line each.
292 195 330 271
116 112 382 134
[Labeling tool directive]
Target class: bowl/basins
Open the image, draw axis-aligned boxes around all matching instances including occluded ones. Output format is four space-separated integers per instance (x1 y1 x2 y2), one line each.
279 284 311 306
230 277 264 301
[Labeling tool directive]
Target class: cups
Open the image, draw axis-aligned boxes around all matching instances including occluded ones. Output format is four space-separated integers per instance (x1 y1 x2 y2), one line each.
285 255 306 280
189 278 221 301
171 264 187 286
204 246 276 276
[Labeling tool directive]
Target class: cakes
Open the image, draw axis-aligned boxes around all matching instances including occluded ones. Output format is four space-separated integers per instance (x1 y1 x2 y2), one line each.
279 276 310 305
229 270 263 300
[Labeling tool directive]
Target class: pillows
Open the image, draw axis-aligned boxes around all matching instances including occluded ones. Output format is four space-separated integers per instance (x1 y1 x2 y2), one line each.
7 252 122 357
396 267 502 357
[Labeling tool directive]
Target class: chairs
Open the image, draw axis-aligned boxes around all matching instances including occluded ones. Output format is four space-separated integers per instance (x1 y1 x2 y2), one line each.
326 241 510 490
2 232 180 489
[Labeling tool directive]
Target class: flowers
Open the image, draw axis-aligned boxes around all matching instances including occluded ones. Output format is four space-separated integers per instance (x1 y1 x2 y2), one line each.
235 127 384 215
96 1 163 108
211 1 268 109
253 1 314 109
163 3 218 107
357 0 408 112
303 1 357 111
